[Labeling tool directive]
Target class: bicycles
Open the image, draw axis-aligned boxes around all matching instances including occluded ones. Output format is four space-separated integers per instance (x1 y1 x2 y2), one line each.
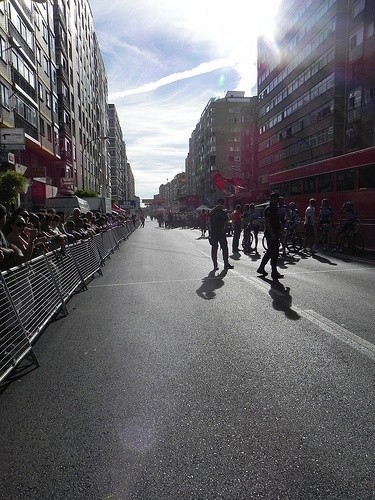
242 217 258 253
262 217 365 256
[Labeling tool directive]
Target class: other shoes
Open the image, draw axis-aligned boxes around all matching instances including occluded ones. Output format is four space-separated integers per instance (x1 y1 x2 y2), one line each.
303 244 344 253
214 264 219 270
233 244 254 253
224 263 234 269
0 299 52 366
271 270 284 278
257 267 269 275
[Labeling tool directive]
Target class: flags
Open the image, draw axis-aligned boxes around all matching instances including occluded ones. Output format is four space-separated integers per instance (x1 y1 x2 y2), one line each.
212 172 246 193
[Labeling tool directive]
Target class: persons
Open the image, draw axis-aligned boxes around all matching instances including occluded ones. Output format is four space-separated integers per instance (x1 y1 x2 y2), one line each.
150 209 210 239
0 204 138 375
303 198 318 253
256 192 284 278
243 204 260 252
334 201 357 253
277 196 302 256
318 198 336 251
209 199 234 270
140 214 145 228
231 205 243 252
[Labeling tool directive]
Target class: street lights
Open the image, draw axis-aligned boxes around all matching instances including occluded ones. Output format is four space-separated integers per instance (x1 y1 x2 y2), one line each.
81 136 110 191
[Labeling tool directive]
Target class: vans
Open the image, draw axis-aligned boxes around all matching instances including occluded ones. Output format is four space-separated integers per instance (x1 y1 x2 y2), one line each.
44 196 90 220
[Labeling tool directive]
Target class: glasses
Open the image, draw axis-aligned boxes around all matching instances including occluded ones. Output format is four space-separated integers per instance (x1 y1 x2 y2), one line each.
15 221 27 227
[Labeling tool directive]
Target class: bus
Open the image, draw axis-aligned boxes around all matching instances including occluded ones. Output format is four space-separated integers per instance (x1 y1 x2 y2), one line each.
268 145 375 253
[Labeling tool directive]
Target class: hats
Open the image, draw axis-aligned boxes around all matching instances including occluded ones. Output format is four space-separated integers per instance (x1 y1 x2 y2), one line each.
217 199 224 206
271 192 280 199
73 209 82 215
